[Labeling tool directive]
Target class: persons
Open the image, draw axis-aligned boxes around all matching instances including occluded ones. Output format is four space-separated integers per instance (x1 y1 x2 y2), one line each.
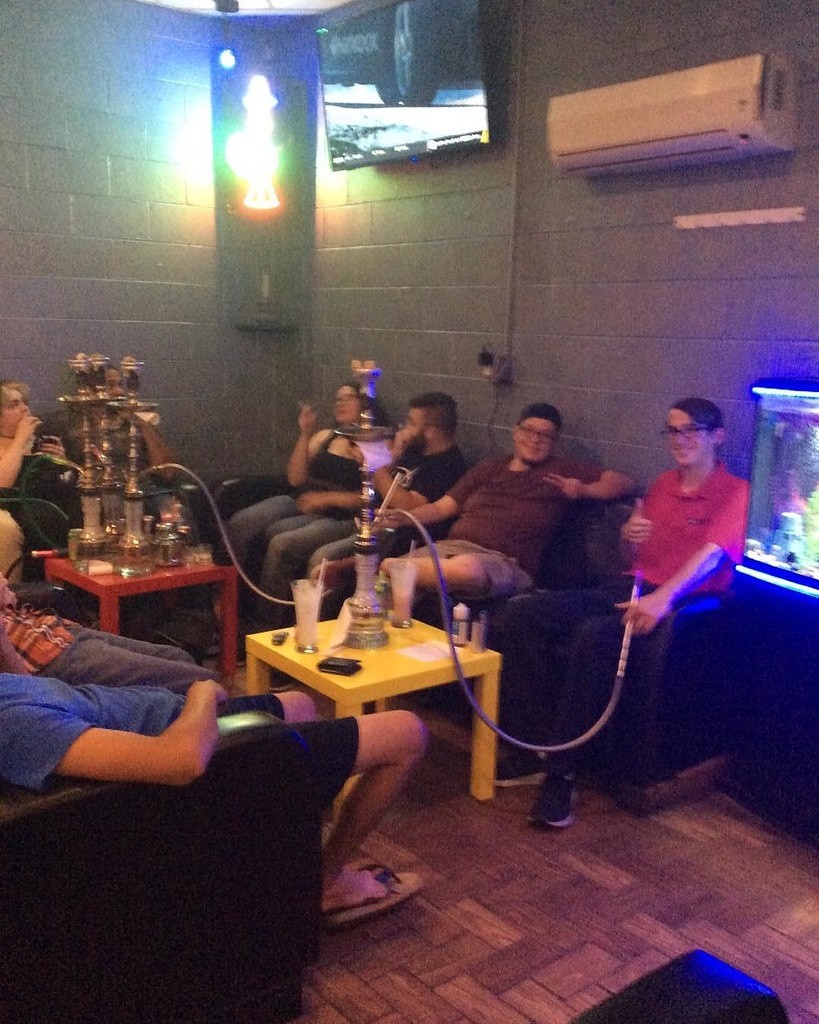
209 381 395 656
236 392 464 692
488 397 751 829
0 365 423 929
375 403 635 619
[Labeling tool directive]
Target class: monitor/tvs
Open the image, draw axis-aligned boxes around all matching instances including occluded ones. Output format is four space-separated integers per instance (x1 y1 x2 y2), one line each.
316 0 502 173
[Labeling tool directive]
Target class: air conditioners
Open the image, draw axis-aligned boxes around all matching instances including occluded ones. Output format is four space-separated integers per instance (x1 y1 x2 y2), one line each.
547 49 793 172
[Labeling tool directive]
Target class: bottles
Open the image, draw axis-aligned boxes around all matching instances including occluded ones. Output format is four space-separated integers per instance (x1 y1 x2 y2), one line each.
153 523 183 565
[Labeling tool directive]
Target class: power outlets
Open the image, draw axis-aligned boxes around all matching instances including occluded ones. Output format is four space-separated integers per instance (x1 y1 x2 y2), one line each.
482 354 507 387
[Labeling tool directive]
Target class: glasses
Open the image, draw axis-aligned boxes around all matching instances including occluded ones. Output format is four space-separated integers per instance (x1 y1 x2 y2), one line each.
660 423 710 439
519 426 559 442
335 394 362 406
403 419 434 429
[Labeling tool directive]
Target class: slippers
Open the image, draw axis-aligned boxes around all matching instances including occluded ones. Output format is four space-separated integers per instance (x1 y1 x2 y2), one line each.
320 865 422 929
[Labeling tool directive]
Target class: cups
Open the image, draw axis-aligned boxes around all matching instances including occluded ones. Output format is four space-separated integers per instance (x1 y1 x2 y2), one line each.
388 564 417 628
452 617 468 648
291 579 326 653
198 543 212 564
88 560 113 575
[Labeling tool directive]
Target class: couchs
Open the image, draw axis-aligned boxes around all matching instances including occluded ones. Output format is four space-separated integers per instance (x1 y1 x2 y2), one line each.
0 580 325 1024
211 469 726 809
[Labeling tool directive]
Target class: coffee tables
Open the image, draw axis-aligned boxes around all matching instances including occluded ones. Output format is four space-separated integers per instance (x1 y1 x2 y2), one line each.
50 528 243 664
243 612 494 817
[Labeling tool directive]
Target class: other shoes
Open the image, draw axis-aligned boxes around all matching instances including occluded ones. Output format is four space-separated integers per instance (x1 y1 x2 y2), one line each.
494 754 543 786
269 673 295 691
207 635 222 653
237 651 249 666
528 773 580 828
